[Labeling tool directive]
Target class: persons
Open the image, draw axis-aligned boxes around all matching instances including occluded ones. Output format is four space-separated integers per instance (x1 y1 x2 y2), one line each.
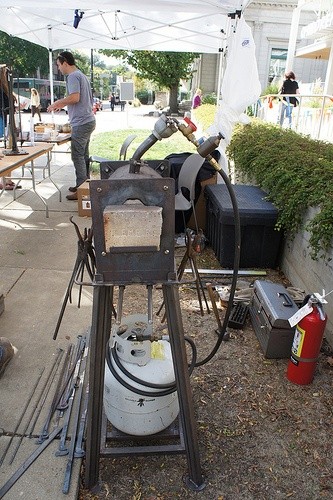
277 71 299 129
120 101 126 111
110 93 115 111
0 64 27 190
193 89 203 109
29 88 41 123
47 51 96 200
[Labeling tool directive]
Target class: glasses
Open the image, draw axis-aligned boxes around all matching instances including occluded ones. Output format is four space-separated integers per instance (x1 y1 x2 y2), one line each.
56 53 67 63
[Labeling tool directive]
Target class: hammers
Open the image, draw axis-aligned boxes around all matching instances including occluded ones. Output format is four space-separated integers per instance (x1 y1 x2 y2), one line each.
206 283 229 339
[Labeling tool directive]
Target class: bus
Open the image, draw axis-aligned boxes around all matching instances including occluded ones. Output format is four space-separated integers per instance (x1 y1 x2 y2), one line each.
11 77 67 113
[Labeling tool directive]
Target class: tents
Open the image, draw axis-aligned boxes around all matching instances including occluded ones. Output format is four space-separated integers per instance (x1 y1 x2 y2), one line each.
0 0 251 137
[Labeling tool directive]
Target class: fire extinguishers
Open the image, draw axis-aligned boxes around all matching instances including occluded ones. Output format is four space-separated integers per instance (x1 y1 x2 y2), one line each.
287 289 333 384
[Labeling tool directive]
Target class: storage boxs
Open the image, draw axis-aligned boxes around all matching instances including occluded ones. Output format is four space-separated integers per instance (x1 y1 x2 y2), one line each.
35 125 55 133
203 183 288 271
76 178 94 217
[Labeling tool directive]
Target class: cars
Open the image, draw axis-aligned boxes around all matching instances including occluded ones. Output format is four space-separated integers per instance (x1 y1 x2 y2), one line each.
93 98 103 111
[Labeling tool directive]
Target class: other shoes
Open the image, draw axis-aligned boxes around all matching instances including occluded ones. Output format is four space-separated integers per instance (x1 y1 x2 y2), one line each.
66 191 78 200
0 184 14 190
5 182 21 188
68 187 77 192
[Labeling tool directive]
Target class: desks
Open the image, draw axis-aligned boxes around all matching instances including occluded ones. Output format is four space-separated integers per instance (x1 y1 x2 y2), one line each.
0 141 55 218
13 132 72 203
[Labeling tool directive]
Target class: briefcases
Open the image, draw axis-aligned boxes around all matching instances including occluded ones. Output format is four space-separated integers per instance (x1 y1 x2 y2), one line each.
248 280 300 359
